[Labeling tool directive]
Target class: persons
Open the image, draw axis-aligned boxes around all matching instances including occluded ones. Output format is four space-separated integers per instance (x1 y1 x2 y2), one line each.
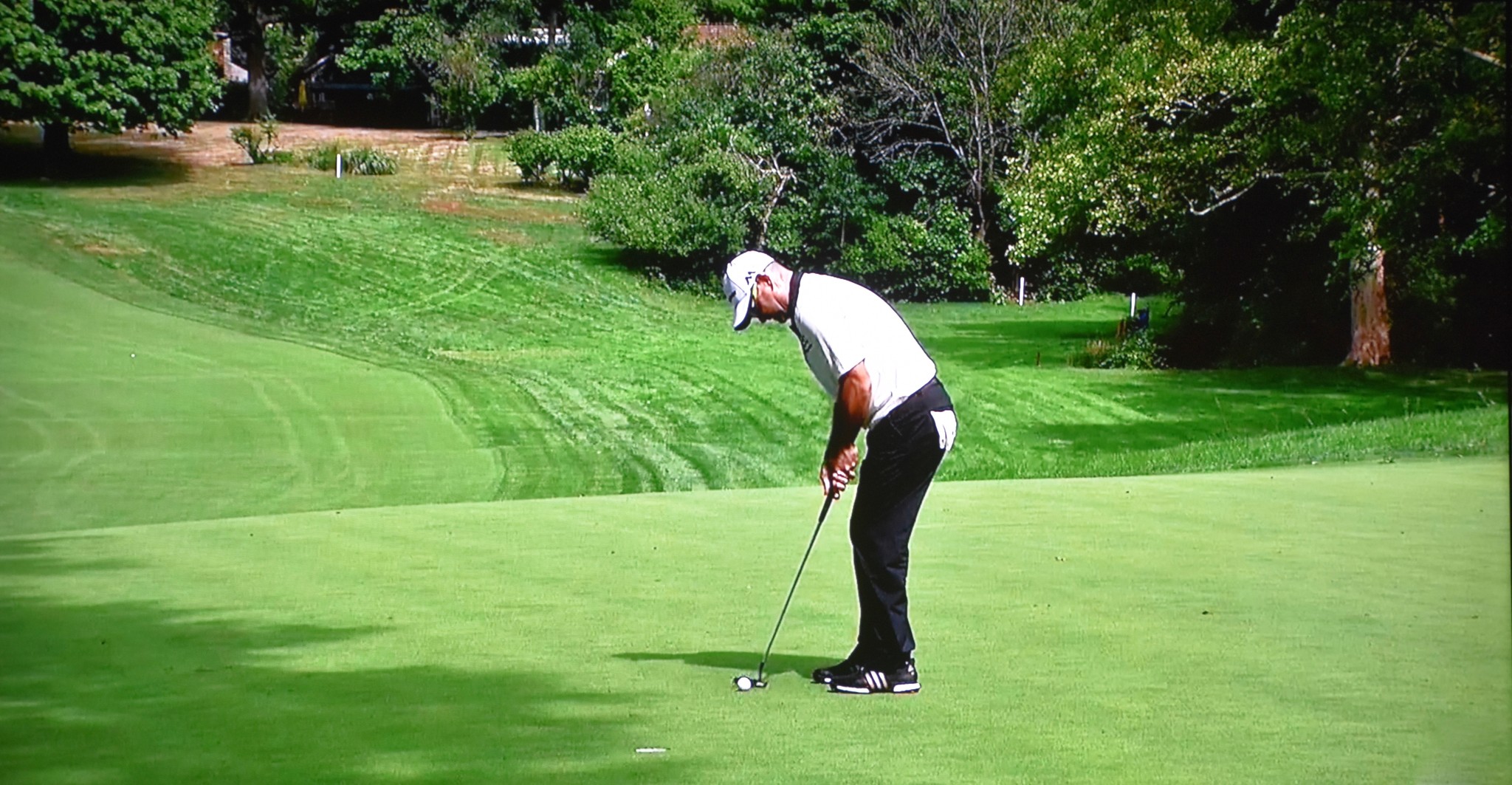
720 249 958 694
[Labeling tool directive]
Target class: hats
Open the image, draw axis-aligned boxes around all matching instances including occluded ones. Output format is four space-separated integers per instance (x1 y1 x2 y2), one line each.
721 250 776 330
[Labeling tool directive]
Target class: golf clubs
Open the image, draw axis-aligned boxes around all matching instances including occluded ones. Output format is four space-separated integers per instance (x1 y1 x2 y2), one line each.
734 482 842 688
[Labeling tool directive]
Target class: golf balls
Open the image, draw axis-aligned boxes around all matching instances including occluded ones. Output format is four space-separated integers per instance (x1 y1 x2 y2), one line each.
737 675 751 692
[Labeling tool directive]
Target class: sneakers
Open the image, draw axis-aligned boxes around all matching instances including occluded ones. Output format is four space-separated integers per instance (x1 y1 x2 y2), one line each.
829 665 920 694
812 659 864 684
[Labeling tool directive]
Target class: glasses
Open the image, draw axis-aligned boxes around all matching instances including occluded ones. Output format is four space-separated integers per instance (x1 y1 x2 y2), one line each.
749 281 758 318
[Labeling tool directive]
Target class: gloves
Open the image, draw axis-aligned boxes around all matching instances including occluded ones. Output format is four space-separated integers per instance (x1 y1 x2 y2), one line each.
930 409 957 451
